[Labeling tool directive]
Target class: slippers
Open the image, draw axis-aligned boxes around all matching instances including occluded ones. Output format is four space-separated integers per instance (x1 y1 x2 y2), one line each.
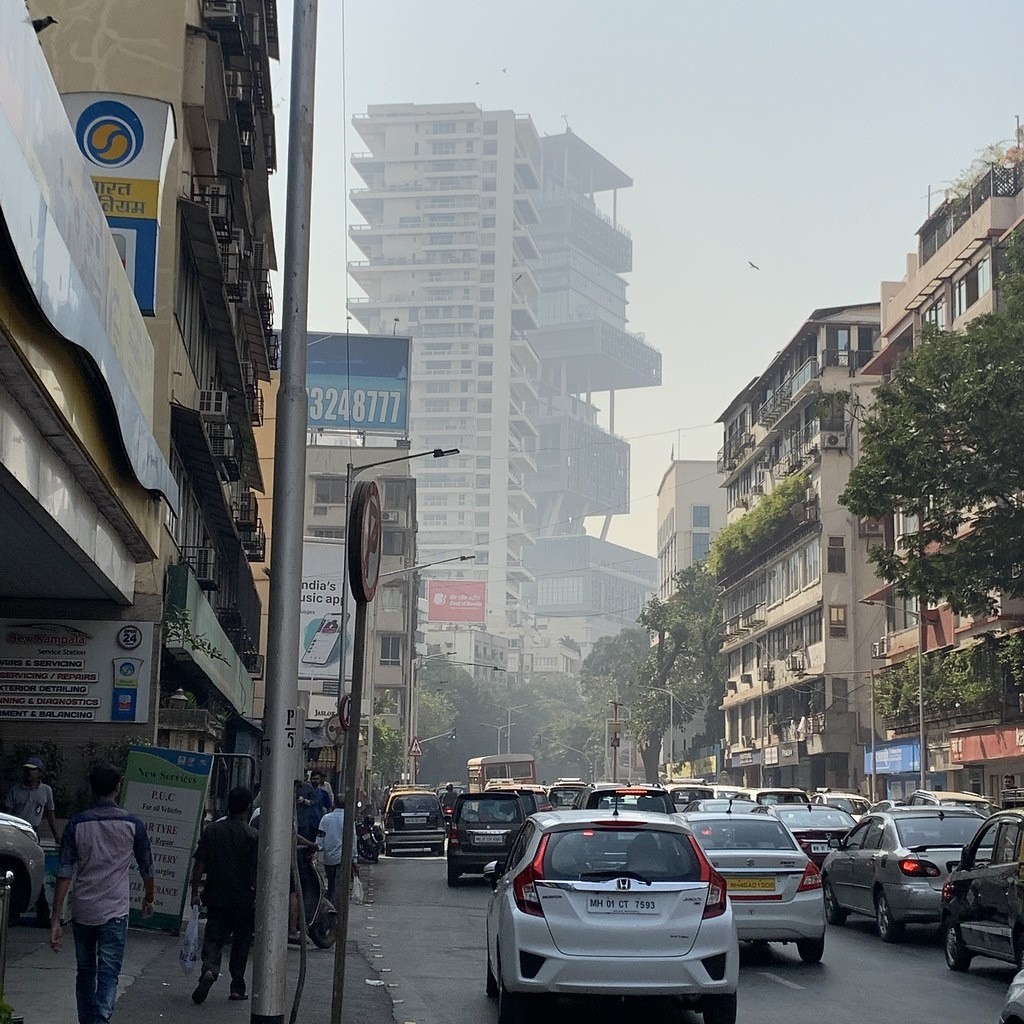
192 973 215 1003
229 992 249 1001
289 931 310 945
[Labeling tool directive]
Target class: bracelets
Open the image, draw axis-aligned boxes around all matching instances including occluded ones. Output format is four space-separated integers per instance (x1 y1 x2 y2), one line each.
143 896 155 906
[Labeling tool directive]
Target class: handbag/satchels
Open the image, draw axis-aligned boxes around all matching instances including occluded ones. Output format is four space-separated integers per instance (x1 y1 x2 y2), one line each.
179 902 199 979
350 874 364 906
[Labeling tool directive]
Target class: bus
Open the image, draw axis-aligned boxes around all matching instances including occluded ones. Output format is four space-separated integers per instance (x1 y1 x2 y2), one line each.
466 754 536 792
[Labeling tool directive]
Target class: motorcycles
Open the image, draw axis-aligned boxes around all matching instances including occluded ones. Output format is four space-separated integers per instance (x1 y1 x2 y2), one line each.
356 801 386 864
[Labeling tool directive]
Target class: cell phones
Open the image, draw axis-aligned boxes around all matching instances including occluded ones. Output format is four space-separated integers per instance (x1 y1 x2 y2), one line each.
301 613 350 666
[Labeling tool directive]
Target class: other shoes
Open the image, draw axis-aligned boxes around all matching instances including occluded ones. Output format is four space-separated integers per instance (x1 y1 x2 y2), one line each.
37 912 50 925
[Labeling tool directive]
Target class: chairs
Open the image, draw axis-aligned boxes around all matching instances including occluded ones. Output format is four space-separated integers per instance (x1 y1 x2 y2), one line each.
625 846 668 873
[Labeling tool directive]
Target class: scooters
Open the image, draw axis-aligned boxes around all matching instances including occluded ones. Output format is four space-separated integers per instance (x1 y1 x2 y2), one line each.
908 789 994 818
288 830 339 948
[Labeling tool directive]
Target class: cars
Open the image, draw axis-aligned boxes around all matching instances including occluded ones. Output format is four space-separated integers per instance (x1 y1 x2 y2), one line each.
820 811 998 944
380 777 978 888
0 811 45 921
998 969 1024 1024
481 808 738 1024
671 813 826 963
941 807 1024 971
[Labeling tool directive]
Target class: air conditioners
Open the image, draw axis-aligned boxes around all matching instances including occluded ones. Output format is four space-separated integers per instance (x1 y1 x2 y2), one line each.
759 664 775 682
181 0 279 558
720 738 727 749
861 518 885 534
380 510 398 523
785 652 798 671
871 641 885 656
822 430 848 450
741 736 751 749
729 604 766 634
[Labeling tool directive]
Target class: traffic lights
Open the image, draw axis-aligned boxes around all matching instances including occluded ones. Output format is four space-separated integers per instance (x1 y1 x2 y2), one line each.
451 727 456 740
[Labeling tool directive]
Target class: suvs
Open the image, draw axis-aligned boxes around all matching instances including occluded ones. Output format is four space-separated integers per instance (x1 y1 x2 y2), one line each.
992 788 1024 865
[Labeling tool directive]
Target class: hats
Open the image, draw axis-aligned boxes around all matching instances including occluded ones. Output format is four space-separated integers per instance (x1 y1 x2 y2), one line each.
23 757 43 770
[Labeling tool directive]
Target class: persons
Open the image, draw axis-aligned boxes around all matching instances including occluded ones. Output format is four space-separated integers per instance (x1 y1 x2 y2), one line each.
4 757 61 847
1001 774 1024 810
249 770 360 902
191 787 259 1004
439 784 457 808
49 764 154 1024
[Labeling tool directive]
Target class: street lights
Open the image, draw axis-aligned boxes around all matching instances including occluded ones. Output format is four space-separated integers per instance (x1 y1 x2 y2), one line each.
476 722 518 755
792 600 925 802
366 555 506 803
336 448 460 791
487 703 529 753
625 681 674 778
